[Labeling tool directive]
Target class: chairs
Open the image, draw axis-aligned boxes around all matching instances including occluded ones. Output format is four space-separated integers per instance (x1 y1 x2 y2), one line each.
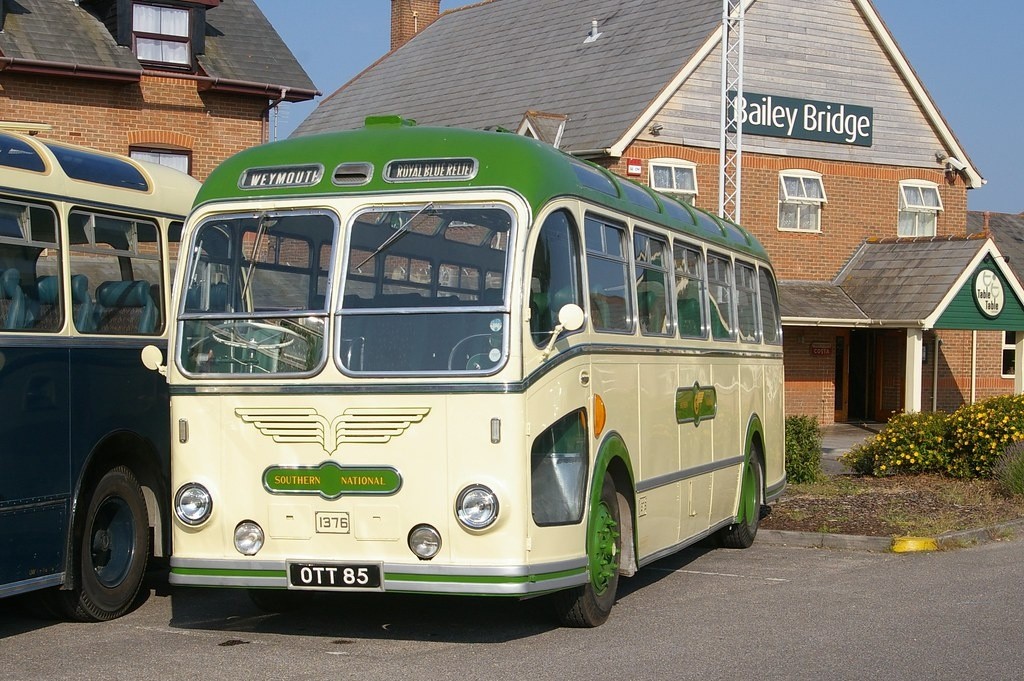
0 266 766 374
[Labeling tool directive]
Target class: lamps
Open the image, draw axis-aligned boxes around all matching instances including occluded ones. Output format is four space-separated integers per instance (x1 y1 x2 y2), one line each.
648 123 664 137
984 256 1010 263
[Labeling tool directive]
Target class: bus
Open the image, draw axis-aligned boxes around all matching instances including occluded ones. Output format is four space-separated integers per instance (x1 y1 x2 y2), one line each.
138 114 790 633
0 127 212 623
